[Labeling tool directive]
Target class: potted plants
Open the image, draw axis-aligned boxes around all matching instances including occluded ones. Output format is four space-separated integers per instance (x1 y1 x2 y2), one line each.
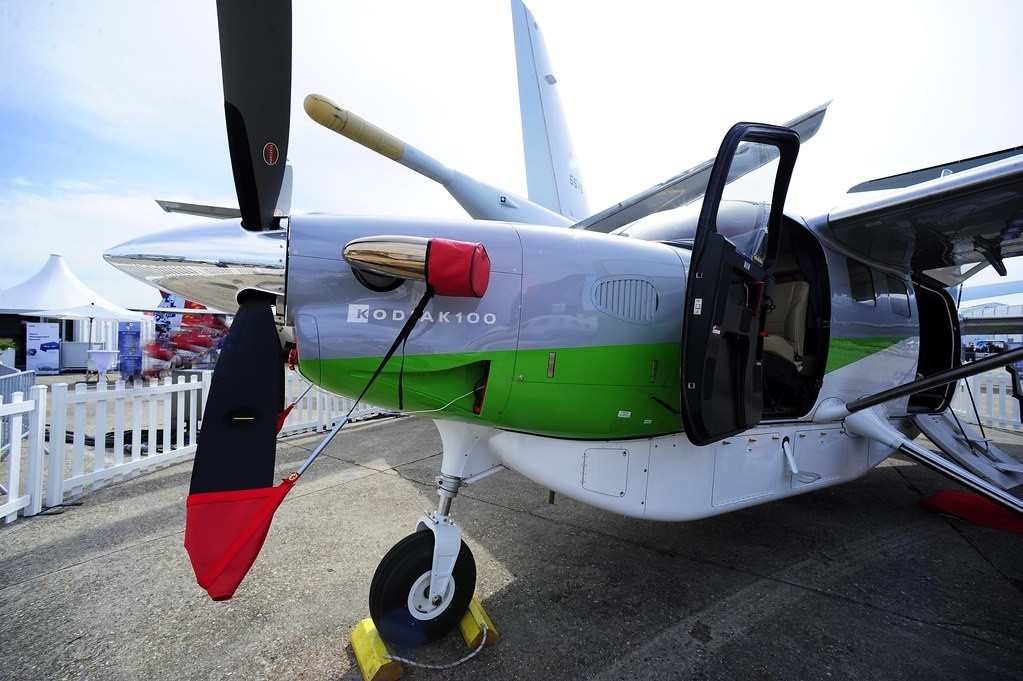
0 341 15 367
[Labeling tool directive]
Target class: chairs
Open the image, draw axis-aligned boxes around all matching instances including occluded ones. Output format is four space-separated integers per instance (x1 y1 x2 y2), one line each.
761 281 810 417
85 349 121 385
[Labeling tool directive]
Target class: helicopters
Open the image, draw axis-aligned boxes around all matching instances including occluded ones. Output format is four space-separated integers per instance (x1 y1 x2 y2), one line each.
100 0 1023 650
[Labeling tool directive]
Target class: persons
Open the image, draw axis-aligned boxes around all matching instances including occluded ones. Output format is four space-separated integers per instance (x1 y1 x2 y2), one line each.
967 345 988 361
985 344 994 353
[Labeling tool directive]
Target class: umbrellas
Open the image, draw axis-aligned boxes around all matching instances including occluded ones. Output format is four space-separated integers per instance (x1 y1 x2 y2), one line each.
20 304 154 371
